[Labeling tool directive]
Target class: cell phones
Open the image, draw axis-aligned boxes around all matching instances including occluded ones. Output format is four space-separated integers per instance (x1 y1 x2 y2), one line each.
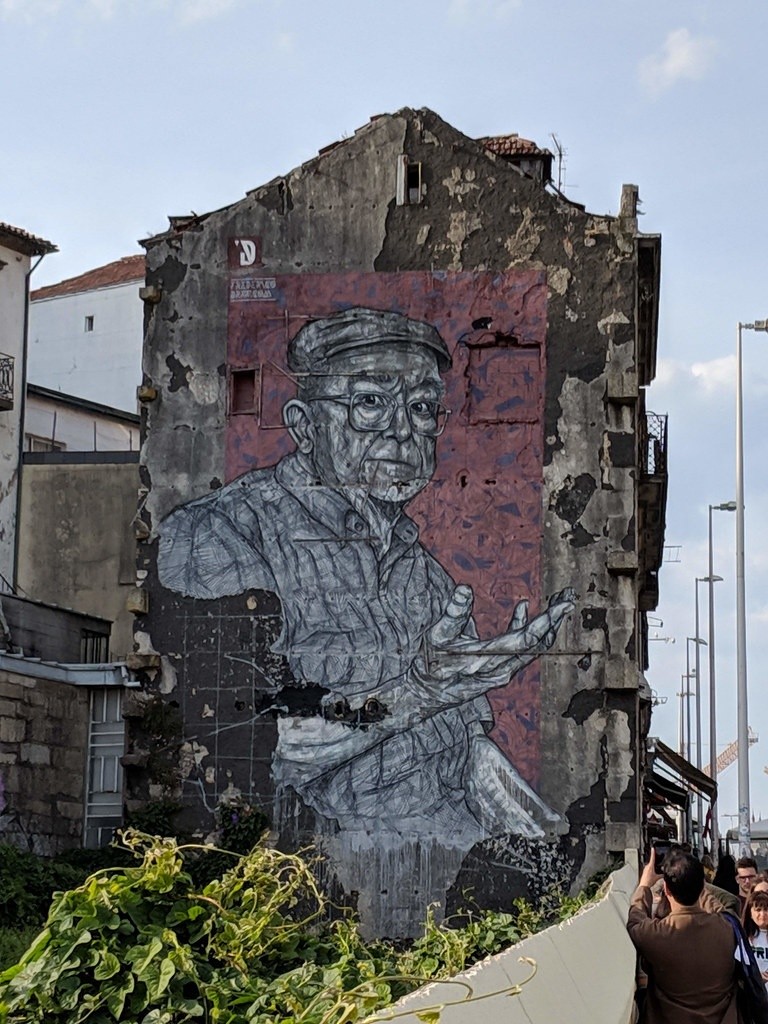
654 840 671 874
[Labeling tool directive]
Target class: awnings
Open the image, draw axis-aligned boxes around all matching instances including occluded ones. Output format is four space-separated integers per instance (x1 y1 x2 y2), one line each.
643 738 718 825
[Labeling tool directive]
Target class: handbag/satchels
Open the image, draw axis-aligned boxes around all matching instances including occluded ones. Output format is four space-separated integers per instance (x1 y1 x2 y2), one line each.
721 911 768 1024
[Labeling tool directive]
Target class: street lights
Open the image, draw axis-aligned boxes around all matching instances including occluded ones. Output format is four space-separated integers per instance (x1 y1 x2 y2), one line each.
707 500 747 867
694 574 726 860
734 319 768 860
678 672 698 846
685 637 708 843
675 690 696 757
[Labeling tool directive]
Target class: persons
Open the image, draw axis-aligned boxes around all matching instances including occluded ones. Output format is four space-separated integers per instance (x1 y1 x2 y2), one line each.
627 840 768 1024
